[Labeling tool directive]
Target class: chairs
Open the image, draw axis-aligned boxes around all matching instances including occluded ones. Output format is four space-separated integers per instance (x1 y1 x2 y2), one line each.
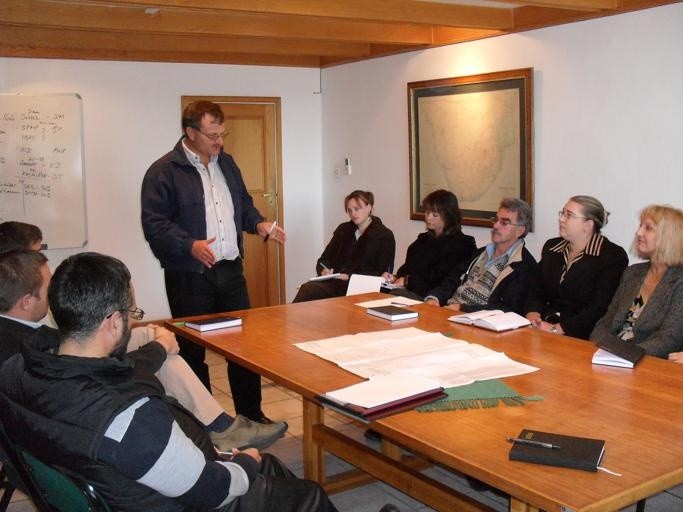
0 439 115 512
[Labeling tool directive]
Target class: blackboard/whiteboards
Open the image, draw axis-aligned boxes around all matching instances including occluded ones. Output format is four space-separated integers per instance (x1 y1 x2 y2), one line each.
1 93 87 250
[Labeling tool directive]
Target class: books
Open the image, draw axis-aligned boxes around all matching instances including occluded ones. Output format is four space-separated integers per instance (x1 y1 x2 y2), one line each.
508 428 623 478
365 305 420 322
590 334 648 369
448 308 532 334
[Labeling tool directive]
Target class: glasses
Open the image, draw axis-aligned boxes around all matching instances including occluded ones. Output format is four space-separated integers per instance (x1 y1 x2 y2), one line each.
106 307 145 321
489 217 526 228
194 127 230 139
558 211 589 220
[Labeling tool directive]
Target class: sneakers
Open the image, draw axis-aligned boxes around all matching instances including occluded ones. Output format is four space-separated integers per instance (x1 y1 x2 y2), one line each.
208 413 289 454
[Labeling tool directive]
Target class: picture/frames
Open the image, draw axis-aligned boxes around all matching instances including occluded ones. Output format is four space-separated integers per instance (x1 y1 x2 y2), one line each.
407 67 534 232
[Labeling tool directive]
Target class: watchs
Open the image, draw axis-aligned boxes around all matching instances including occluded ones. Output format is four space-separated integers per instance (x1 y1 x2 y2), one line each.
549 323 558 334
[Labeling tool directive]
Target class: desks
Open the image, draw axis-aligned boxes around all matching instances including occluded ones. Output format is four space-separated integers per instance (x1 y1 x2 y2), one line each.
163 292 683 512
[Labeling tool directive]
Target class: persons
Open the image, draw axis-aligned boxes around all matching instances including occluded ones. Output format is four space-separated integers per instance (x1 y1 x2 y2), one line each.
141 100 288 439
291 190 396 303
516 195 628 341
422 196 537 317
381 188 476 300
588 203 683 361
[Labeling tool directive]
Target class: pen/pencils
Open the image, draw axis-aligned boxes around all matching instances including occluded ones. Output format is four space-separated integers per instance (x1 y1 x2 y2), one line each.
172 322 185 326
388 262 391 278
263 219 279 242
320 262 332 274
507 438 560 450
